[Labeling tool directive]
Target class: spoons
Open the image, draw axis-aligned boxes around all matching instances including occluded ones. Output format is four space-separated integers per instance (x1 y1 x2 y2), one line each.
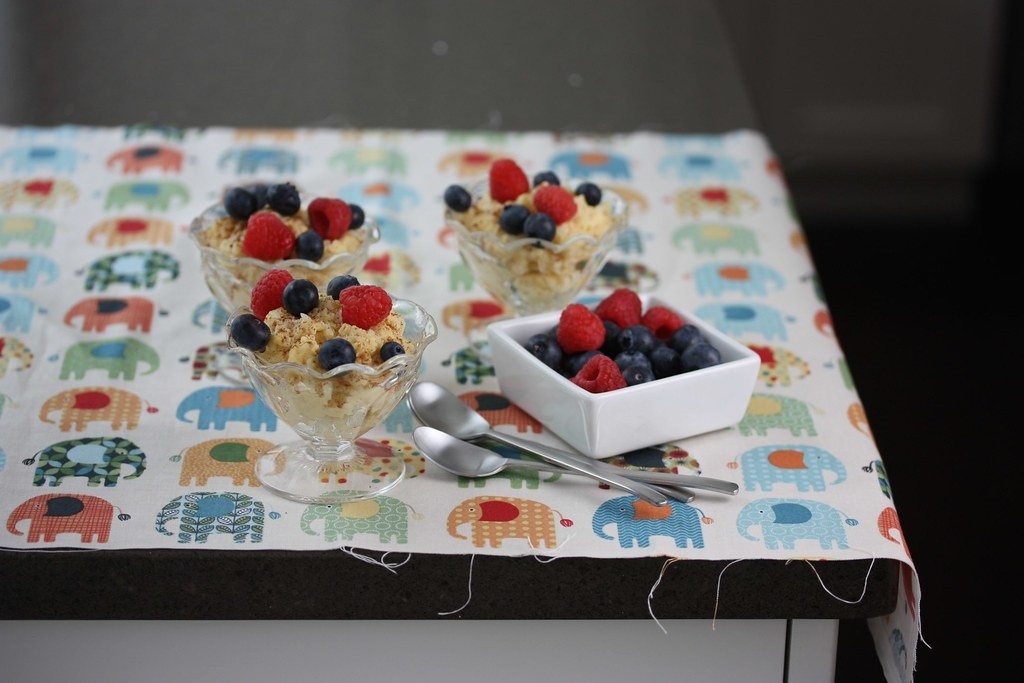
407 380 740 507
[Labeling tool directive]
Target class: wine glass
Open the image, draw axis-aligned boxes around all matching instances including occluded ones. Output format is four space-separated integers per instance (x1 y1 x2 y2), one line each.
225 283 438 505
186 193 381 386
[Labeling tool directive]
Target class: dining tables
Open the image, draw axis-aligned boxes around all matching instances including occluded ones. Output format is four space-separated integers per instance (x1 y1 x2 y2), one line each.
0 121 899 683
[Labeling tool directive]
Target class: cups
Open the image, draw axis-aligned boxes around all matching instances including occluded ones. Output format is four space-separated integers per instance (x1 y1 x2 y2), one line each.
434 171 631 364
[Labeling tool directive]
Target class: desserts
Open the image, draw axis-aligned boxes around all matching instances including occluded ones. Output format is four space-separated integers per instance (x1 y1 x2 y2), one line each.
444 159 624 314
228 267 420 445
195 179 369 318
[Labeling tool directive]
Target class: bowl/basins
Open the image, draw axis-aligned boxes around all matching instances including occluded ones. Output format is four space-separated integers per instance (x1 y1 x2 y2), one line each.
487 297 760 460
